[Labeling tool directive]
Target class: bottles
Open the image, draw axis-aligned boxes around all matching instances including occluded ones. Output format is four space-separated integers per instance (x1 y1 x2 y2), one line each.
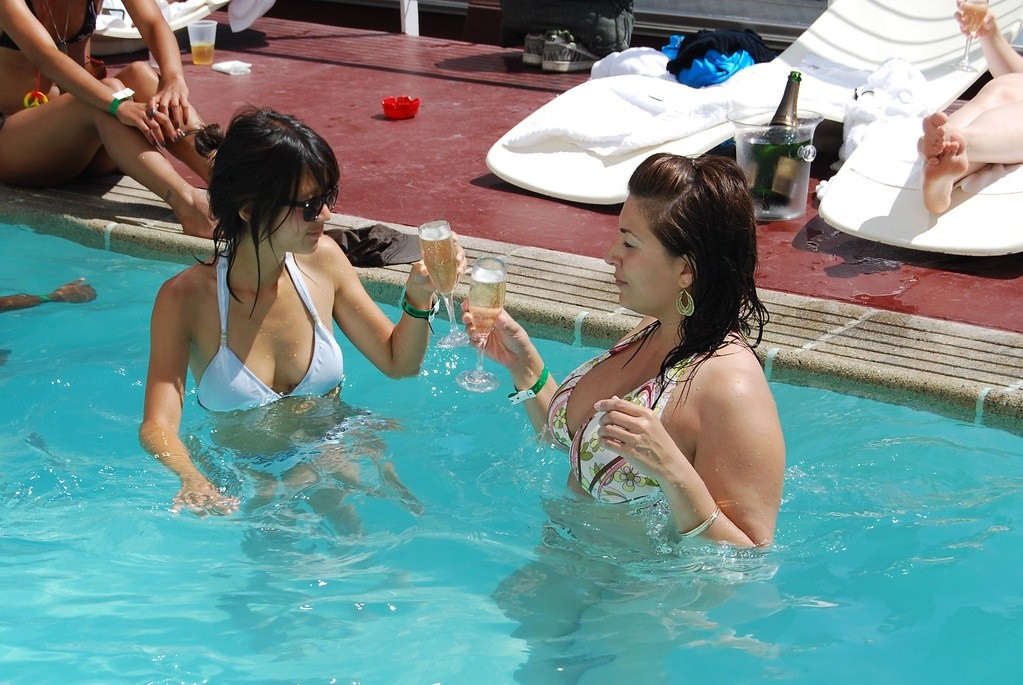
754 73 806 204
148 0 171 70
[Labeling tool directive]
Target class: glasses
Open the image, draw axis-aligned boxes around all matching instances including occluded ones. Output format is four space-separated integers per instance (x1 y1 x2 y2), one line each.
285 191 332 223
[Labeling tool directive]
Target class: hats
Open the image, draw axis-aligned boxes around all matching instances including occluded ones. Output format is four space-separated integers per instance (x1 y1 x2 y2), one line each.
323 223 423 267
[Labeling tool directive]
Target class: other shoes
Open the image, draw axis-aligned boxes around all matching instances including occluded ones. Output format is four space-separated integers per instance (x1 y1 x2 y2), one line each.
523 29 599 71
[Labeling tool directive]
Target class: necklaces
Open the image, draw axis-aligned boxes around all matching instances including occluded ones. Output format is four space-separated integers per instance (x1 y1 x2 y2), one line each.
45 0 72 55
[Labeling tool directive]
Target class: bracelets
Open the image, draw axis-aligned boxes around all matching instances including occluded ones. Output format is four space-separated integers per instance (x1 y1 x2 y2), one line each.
402 286 441 324
109 88 135 117
41 295 50 302
676 503 721 540
507 363 549 405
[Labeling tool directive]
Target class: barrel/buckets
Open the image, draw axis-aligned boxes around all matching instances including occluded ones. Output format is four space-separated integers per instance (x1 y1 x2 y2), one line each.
727 107 825 220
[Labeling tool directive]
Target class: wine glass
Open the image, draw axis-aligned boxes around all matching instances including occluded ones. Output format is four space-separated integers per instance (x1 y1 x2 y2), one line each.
456 257 505 392
947 0 987 72
419 221 472 349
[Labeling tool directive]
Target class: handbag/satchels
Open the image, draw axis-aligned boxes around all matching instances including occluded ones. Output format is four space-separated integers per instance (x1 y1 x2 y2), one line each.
500 0 635 57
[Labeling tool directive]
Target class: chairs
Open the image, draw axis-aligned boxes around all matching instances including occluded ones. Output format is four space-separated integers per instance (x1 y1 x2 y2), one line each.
484 0 1023 258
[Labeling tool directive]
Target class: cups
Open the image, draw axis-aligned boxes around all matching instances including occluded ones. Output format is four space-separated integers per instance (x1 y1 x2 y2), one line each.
186 20 218 65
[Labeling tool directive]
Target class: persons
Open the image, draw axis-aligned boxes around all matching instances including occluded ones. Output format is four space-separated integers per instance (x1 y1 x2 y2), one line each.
460 152 787 545
0 279 97 313
917 0 1023 214
0 0 232 241
136 105 470 422
